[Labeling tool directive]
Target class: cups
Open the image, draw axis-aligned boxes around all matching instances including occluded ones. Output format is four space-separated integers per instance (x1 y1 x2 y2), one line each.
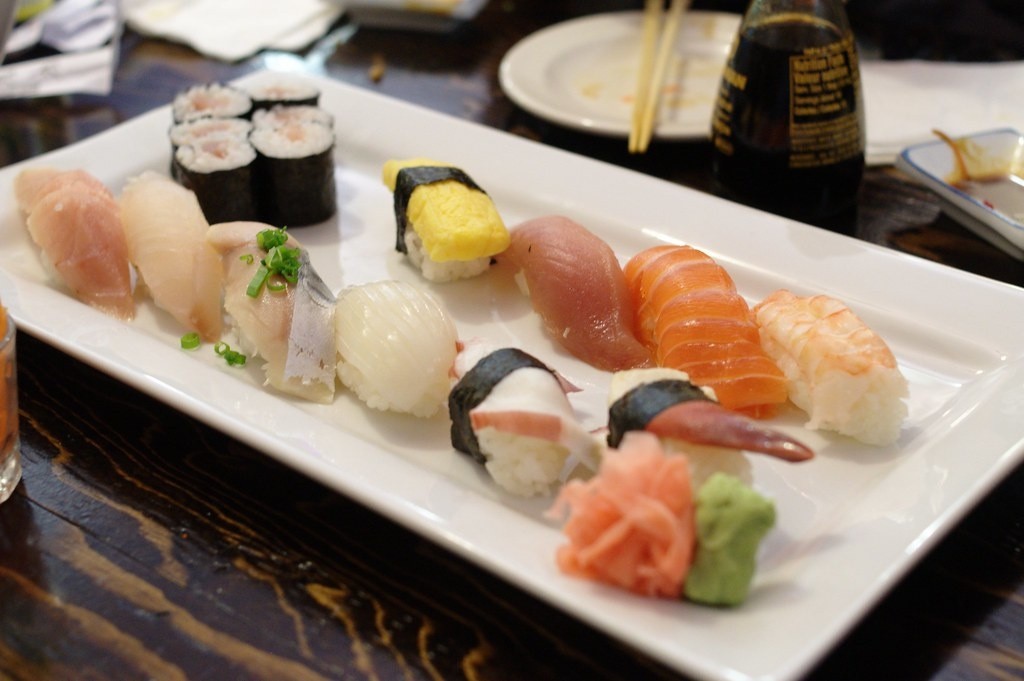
0 312 22 503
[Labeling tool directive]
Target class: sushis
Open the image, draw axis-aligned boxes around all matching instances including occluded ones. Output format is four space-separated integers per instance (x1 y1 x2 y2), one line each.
11 76 913 495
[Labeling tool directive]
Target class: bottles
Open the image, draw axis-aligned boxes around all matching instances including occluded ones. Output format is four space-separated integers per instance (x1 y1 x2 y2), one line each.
711 0 867 237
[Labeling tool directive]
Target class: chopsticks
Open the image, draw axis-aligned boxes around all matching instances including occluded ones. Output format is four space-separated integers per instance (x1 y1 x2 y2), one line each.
630 0 684 153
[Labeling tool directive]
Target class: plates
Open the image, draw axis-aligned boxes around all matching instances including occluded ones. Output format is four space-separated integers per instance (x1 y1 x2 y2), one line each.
897 126 1023 250
0 68 1024 681
498 13 741 139
